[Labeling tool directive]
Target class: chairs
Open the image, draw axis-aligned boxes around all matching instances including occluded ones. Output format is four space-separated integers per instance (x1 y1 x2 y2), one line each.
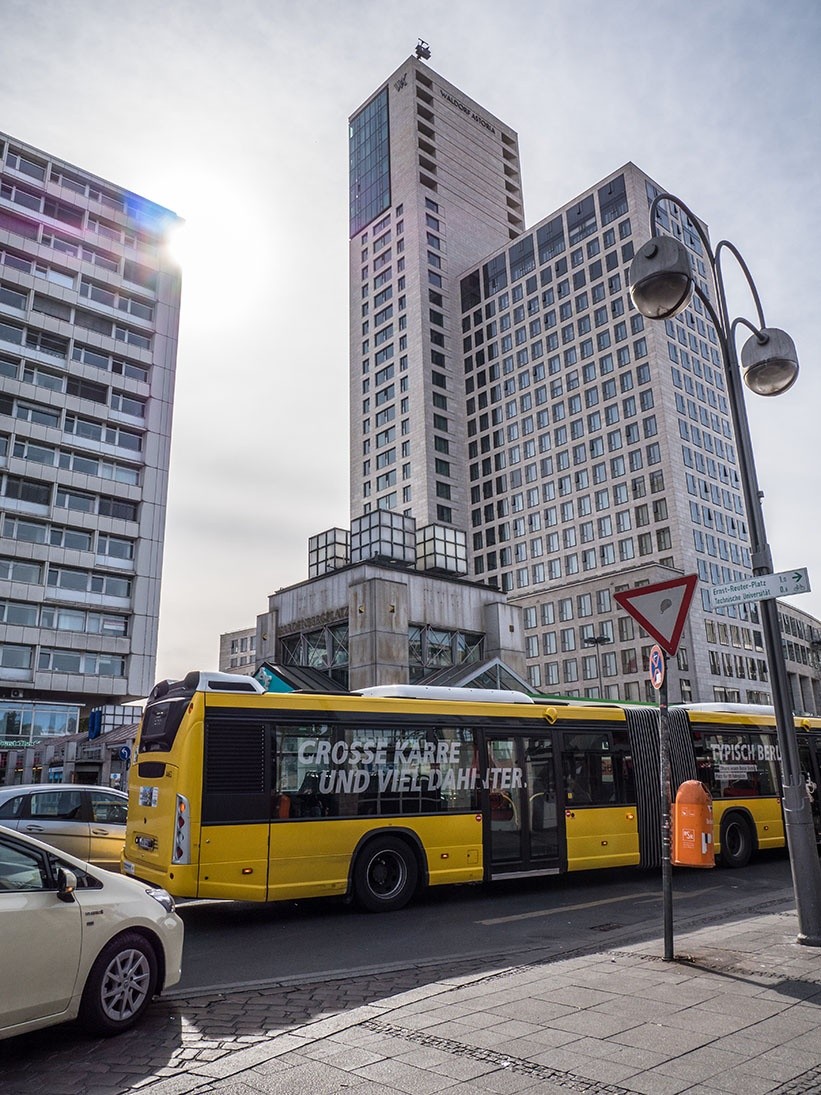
290 765 771 822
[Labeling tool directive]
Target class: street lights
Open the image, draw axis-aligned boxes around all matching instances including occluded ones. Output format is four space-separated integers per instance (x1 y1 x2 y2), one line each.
628 193 821 947
584 636 610 698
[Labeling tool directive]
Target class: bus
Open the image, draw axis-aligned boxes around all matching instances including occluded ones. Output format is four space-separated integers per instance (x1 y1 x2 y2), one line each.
120 672 821 913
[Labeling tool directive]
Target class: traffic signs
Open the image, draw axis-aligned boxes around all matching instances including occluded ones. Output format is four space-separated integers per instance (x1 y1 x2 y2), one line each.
708 567 812 608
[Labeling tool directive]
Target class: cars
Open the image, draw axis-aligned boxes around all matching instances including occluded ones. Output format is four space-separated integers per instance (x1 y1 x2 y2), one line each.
0 783 129 874
0 824 185 1041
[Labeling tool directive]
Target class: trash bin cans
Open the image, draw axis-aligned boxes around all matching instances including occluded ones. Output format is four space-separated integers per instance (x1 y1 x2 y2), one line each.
671 780 715 867
